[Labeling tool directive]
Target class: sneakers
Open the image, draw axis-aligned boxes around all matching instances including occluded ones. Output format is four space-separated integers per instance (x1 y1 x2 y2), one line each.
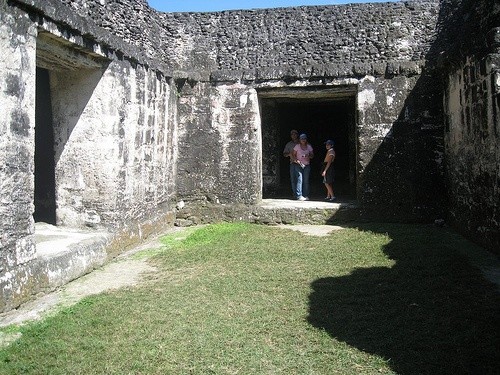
305 196 310 200
297 196 306 201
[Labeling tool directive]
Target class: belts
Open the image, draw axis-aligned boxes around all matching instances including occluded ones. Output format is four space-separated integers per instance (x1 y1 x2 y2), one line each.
305 165 310 166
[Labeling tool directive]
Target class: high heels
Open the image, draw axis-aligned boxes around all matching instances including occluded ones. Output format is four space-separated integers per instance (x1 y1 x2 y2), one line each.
330 196 336 202
324 196 330 200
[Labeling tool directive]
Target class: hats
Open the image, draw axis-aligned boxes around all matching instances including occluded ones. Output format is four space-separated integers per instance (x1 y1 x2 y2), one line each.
291 130 298 134
300 134 307 140
324 140 334 145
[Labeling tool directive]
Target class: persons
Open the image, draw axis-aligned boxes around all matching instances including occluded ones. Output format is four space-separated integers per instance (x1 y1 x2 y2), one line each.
322 140 336 201
292 134 313 201
283 130 301 200
